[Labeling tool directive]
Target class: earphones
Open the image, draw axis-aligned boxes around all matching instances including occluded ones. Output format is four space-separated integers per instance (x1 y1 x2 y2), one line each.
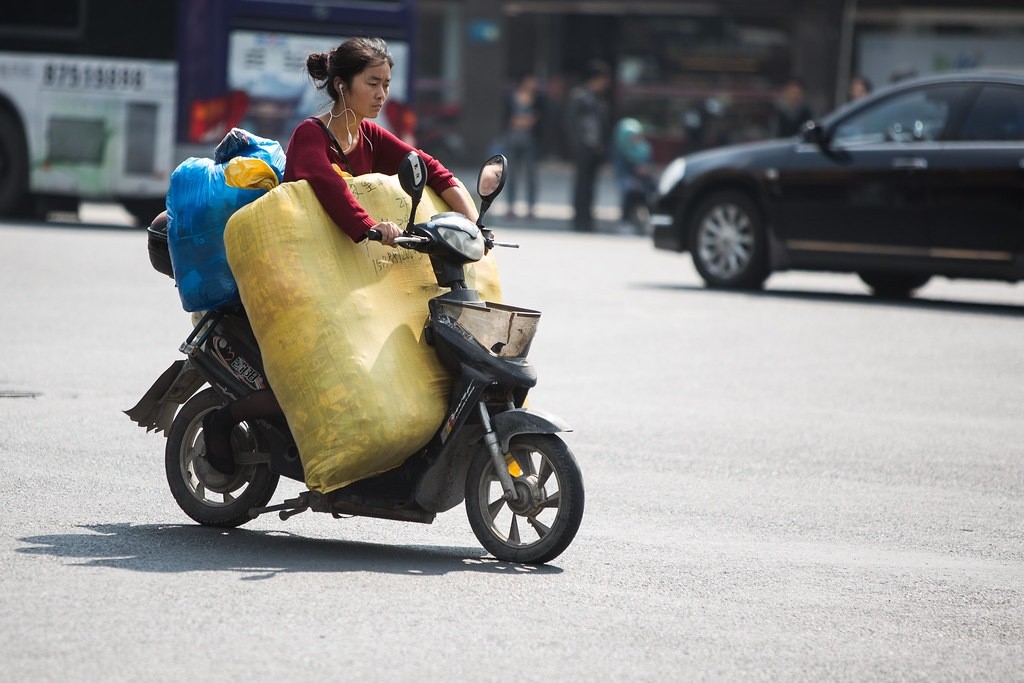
339 83 344 95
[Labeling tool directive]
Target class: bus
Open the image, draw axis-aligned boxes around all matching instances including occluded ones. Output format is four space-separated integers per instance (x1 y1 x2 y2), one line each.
0 0 415 223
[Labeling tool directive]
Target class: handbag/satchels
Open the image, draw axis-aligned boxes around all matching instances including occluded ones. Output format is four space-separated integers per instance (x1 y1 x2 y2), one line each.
165 125 287 312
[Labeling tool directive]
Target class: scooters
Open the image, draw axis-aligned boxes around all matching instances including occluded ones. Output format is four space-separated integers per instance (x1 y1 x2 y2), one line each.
121 150 585 564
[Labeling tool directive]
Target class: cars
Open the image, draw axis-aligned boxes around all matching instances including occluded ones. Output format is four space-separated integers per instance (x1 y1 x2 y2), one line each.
648 72 1024 299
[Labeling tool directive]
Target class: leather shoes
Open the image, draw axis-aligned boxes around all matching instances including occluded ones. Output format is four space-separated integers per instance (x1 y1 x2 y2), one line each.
202 408 236 475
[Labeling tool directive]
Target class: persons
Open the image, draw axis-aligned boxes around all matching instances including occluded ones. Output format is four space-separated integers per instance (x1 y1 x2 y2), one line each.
503 66 875 235
479 156 503 196
202 37 494 475
